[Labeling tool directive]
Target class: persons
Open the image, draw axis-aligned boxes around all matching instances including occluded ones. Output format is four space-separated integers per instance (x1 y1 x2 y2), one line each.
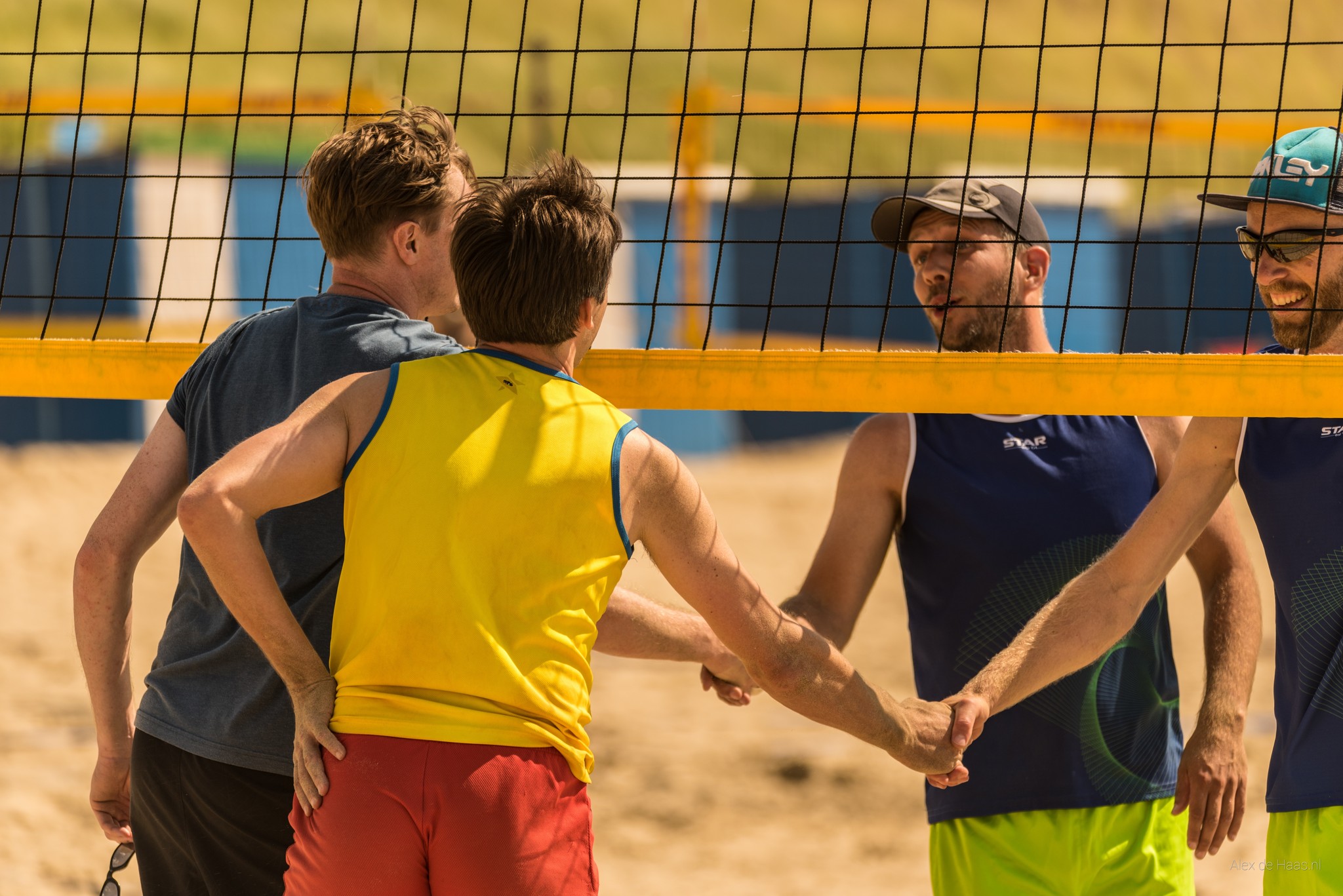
926 134 1343 896
701 181 1263 896
176 157 964 896
72 105 759 896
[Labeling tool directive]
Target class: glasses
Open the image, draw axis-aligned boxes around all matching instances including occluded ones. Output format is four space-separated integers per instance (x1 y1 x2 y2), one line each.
1235 225 1343 263
99 842 137 896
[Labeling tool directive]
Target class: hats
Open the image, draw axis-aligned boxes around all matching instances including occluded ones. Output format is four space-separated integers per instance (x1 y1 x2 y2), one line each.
870 176 1051 254
1197 128 1343 212
